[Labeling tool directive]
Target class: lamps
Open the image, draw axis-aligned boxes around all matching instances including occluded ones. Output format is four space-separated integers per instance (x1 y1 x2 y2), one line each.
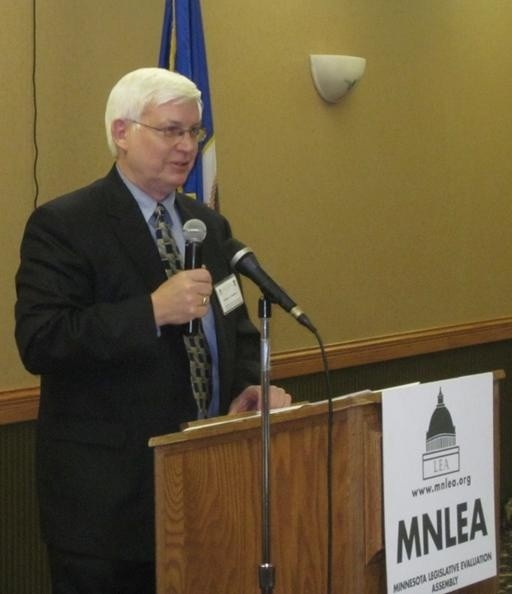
307 52 369 105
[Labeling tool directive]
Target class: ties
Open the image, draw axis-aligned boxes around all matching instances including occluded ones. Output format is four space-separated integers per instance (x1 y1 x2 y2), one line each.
152 204 214 420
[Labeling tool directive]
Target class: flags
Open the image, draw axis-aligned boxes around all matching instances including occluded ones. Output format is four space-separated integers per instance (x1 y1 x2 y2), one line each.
159 0 219 212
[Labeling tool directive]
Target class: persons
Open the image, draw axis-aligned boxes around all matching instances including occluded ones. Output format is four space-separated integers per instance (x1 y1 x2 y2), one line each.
15 66 291 594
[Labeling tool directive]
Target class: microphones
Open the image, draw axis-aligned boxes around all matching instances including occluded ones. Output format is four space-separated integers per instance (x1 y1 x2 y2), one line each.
181 212 209 338
216 237 314 327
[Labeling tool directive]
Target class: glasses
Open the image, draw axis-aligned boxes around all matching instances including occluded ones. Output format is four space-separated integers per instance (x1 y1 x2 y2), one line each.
131 119 208 142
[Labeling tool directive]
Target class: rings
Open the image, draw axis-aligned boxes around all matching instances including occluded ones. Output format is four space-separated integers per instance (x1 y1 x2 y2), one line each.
203 294 208 305
